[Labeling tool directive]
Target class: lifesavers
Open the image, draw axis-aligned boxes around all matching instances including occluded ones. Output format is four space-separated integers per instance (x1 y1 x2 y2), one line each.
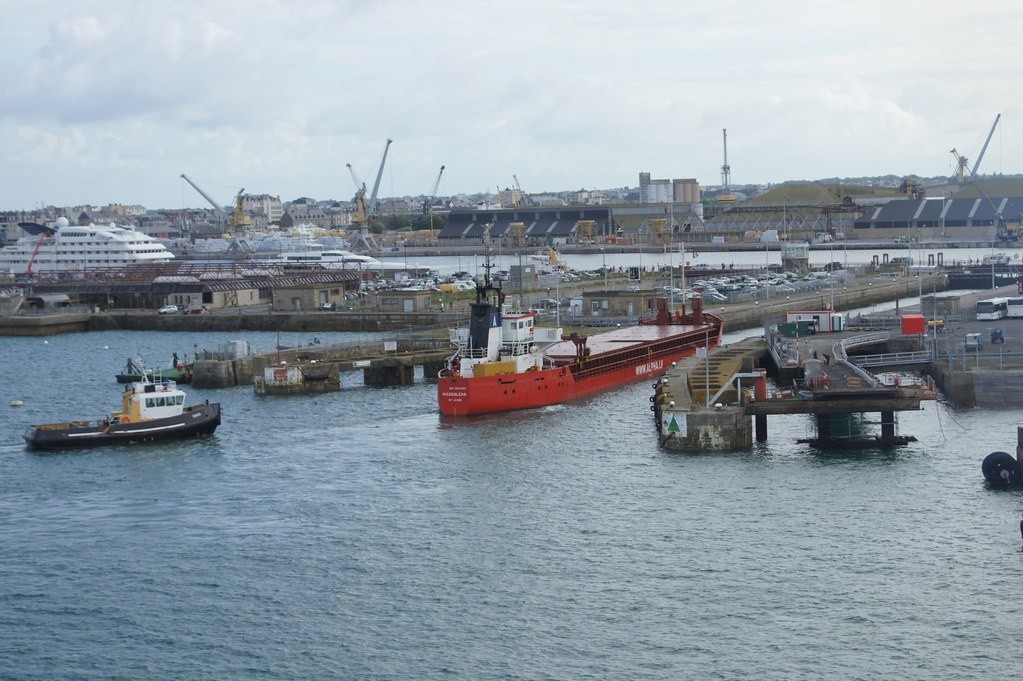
122 417 130 425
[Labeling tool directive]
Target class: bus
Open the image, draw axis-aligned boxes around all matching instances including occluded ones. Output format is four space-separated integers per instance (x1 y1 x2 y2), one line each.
975 296 1012 322
1006 297 1023 318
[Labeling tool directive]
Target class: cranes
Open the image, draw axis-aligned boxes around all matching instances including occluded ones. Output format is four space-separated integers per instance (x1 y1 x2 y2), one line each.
350 137 393 251
951 148 1023 242
423 165 446 214
180 173 255 253
950 112 1001 181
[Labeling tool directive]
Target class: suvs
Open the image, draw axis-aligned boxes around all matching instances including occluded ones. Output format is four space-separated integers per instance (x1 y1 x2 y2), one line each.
690 263 711 270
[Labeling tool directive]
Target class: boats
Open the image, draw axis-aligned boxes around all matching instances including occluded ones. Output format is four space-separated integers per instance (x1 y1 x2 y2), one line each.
22 366 221 448
120 353 197 385
437 221 725 416
531 250 562 262
241 250 430 274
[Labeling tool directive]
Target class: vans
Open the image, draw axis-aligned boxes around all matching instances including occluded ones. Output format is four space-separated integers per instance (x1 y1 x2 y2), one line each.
791 320 819 335
454 280 476 288
890 257 913 267
804 272 827 280
451 283 469 291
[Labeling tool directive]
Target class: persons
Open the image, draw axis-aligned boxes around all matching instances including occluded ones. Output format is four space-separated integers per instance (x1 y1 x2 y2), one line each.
439 298 445 313
454 370 463 382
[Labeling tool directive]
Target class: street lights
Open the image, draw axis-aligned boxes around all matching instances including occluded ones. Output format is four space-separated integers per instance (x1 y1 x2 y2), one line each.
918 228 921 297
922 225 926 266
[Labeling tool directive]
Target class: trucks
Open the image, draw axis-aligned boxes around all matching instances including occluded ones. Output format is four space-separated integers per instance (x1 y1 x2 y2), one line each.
965 333 984 351
895 236 911 243
981 255 1008 267
440 284 459 293
819 235 832 243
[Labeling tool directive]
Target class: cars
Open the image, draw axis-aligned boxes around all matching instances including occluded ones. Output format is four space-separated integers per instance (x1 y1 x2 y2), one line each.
184 305 205 314
764 263 782 270
823 270 839 286
530 299 562 308
579 238 595 245
653 270 800 299
159 305 177 314
825 262 843 270
561 296 583 307
626 286 639 290
345 270 508 300
318 303 337 311
540 268 612 282
